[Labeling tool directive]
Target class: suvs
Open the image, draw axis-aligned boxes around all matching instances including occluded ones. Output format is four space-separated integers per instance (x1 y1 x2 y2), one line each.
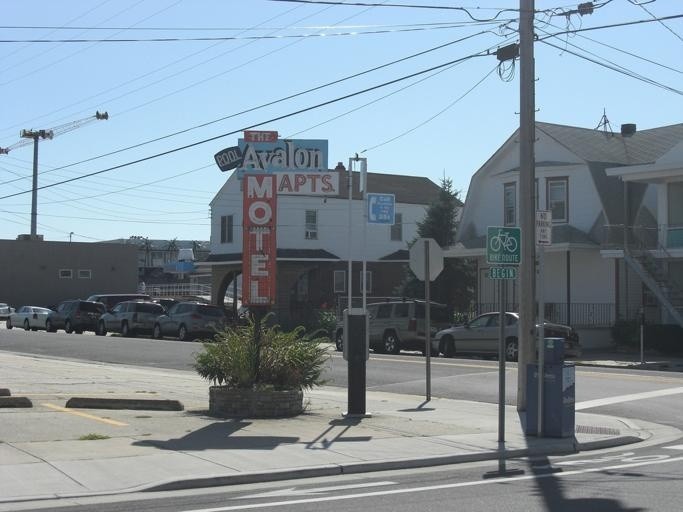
152 299 228 344
335 296 448 361
45 298 106 335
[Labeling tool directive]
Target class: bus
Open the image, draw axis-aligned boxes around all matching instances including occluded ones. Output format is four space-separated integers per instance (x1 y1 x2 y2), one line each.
85 292 151 307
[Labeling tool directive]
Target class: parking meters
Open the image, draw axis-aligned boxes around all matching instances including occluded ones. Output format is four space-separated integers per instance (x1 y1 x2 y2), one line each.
637 305 644 366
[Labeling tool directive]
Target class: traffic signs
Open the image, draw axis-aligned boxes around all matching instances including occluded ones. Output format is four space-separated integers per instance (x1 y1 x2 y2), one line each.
534 211 552 246
490 266 516 279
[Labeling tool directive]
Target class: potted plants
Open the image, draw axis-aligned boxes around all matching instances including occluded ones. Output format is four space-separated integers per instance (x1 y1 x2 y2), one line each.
193 313 333 418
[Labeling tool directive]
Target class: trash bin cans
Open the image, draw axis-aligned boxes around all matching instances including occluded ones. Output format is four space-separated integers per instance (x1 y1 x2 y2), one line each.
524 337 575 438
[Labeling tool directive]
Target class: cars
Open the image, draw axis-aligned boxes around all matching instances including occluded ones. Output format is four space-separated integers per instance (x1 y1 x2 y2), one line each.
0 302 15 320
430 310 583 361
152 298 174 304
3 305 53 331
97 300 163 336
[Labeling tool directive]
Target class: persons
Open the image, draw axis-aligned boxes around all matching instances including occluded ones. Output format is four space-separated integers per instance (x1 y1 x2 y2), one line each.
139 277 146 294
